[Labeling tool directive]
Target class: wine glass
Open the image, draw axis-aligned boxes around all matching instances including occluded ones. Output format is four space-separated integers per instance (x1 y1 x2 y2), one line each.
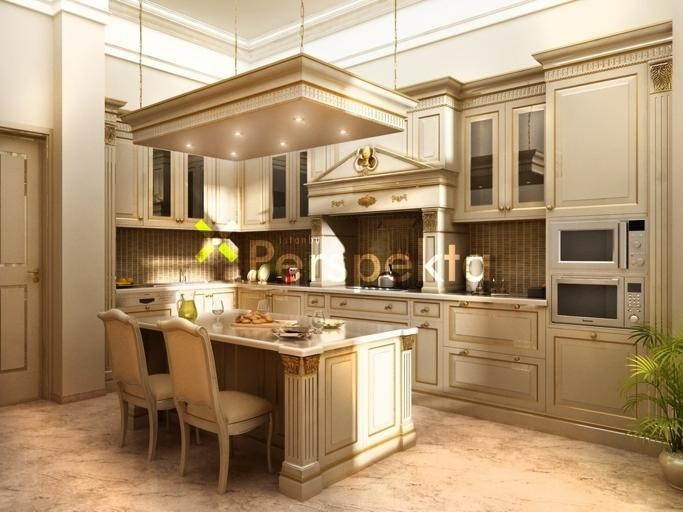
211 300 224 329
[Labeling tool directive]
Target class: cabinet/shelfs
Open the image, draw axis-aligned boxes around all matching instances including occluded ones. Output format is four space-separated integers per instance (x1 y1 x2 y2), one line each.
442 299 547 416
237 153 273 235
412 298 441 397
263 145 317 233
113 119 148 233
544 44 650 223
143 137 211 235
115 288 411 326
211 155 243 234
544 327 653 439
456 67 548 228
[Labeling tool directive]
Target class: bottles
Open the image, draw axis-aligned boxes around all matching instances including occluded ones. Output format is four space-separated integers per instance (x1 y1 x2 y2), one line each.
284 262 290 283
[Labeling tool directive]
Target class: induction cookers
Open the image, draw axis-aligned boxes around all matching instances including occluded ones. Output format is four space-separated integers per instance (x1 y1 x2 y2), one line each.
347 285 409 291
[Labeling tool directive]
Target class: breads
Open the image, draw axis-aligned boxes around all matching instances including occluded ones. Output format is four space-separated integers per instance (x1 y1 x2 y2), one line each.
236 311 273 323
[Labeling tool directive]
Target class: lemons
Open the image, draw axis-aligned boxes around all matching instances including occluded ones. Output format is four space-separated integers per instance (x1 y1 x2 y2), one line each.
120 278 126 282
127 277 132 282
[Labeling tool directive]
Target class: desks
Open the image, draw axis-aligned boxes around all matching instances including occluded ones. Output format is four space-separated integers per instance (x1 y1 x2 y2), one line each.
126 309 422 502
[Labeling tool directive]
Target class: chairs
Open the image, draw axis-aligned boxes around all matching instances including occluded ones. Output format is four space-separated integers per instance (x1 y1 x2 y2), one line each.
98 302 207 459
155 312 281 497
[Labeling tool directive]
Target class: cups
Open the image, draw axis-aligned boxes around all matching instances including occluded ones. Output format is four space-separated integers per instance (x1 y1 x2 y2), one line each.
311 310 325 334
288 268 300 283
256 299 268 312
275 276 282 283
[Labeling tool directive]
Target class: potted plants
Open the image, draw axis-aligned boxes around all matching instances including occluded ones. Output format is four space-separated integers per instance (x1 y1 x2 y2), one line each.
622 320 683 492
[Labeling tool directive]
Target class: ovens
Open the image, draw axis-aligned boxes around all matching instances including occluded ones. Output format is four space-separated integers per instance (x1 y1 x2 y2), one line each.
547 215 647 333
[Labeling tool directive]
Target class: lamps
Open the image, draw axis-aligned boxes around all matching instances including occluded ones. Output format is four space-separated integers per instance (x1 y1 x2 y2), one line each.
122 1 412 165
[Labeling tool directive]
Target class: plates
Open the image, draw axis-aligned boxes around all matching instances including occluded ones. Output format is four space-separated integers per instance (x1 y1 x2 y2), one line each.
270 327 316 340
229 320 297 328
322 320 345 329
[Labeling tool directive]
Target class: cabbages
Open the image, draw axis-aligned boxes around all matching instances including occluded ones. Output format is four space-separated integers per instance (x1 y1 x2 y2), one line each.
247 269 257 281
257 264 271 281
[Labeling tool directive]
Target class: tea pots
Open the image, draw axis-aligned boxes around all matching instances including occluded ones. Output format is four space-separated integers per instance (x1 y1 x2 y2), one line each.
175 291 197 324
377 263 401 288
